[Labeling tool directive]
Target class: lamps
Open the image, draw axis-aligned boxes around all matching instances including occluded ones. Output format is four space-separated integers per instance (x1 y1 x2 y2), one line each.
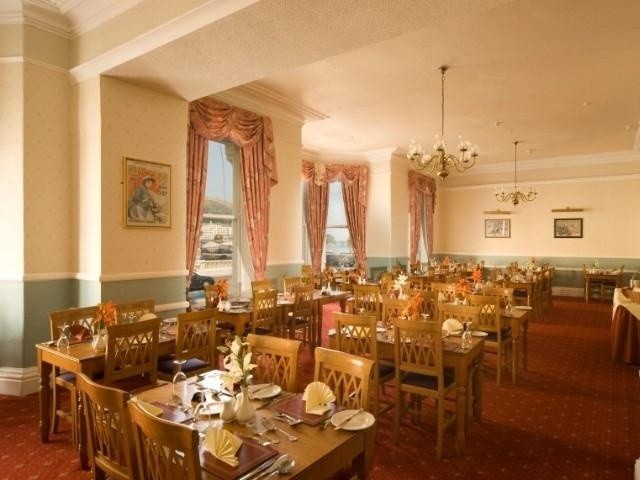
408 63 482 180
493 140 539 206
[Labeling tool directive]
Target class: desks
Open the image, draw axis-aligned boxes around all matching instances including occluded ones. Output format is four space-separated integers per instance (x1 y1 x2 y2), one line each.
582 265 639 364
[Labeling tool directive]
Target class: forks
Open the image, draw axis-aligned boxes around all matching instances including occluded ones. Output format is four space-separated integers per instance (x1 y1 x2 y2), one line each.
261 417 298 441
270 416 304 425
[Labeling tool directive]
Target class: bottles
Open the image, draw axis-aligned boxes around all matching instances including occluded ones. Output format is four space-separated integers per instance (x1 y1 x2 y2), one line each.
221 398 235 425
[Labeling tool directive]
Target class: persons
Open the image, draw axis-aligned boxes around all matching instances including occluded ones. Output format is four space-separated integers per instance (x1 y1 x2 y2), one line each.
128 175 160 222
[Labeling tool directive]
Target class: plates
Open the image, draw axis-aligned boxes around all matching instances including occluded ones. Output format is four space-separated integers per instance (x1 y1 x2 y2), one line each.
470 331 489 337
249 384 283 400
331 410 374 431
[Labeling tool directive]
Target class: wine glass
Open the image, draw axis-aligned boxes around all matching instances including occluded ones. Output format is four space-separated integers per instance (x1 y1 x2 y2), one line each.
195 388 211 432
463 323 472 348
57 324 70 347
173 360 188 393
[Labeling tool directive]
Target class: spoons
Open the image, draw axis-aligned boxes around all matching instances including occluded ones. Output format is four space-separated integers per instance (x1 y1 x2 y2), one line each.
240 454 295 479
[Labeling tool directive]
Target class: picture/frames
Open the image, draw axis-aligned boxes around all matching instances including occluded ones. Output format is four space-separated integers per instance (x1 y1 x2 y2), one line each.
553 218 583 238
123 157 172 228
485 219 510 238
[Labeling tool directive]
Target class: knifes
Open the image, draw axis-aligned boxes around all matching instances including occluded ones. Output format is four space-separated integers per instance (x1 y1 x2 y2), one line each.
335 408 373 431
